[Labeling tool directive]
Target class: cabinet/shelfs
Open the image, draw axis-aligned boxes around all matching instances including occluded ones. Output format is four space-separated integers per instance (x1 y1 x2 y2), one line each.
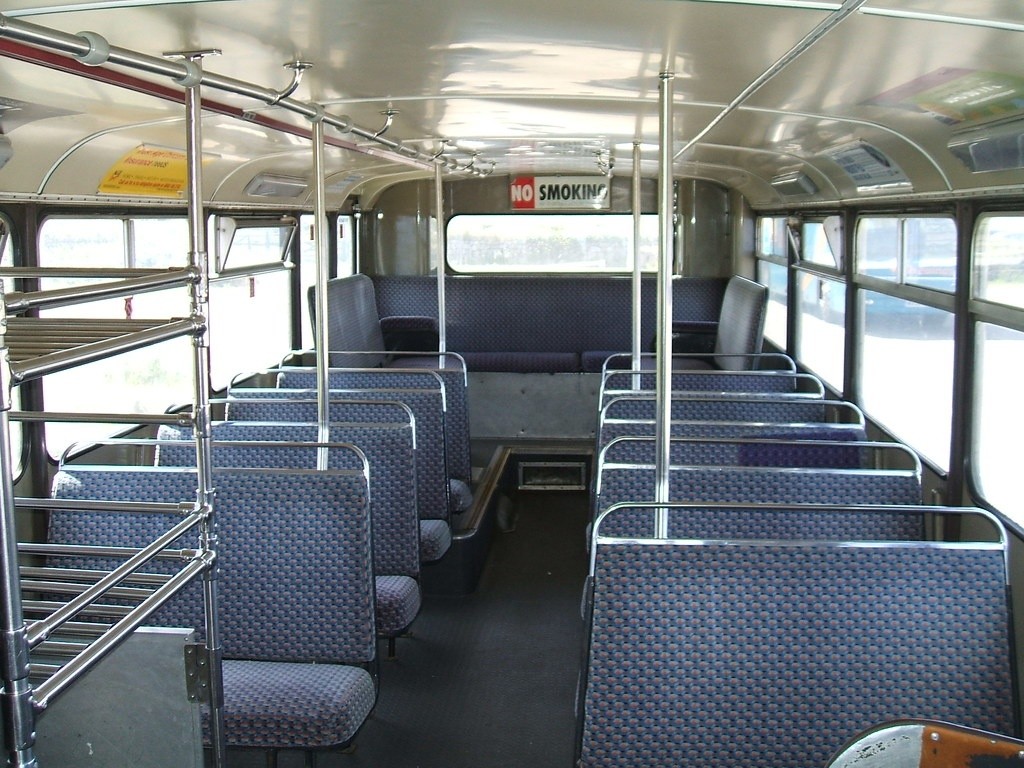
0 318 219 717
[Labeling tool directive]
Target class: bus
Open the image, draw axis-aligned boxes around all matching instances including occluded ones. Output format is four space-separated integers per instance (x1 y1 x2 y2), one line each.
757 216 985 318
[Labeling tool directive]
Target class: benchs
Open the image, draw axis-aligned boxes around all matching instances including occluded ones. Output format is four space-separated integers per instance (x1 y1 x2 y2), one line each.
574 356 1016 768
307 272 771 441
45 350 474 768
